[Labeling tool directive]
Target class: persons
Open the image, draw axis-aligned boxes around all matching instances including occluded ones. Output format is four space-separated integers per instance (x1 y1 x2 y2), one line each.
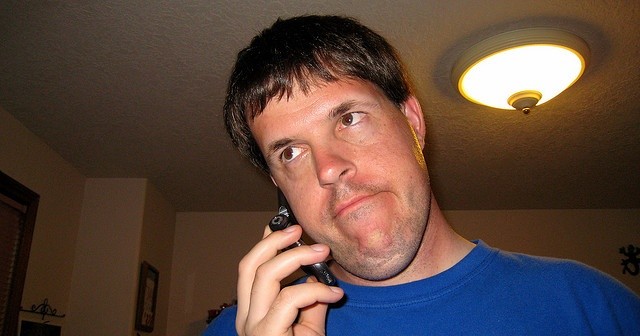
199 14 640 336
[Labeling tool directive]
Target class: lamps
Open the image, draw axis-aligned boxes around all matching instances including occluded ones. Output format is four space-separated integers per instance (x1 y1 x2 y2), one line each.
451 26 592 115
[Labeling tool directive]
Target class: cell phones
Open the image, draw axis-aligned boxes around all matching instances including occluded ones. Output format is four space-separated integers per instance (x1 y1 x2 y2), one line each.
269 189 339 287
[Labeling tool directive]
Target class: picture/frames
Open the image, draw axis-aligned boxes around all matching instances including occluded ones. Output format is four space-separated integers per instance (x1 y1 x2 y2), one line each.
134 259 159 332
0 170 40 336
